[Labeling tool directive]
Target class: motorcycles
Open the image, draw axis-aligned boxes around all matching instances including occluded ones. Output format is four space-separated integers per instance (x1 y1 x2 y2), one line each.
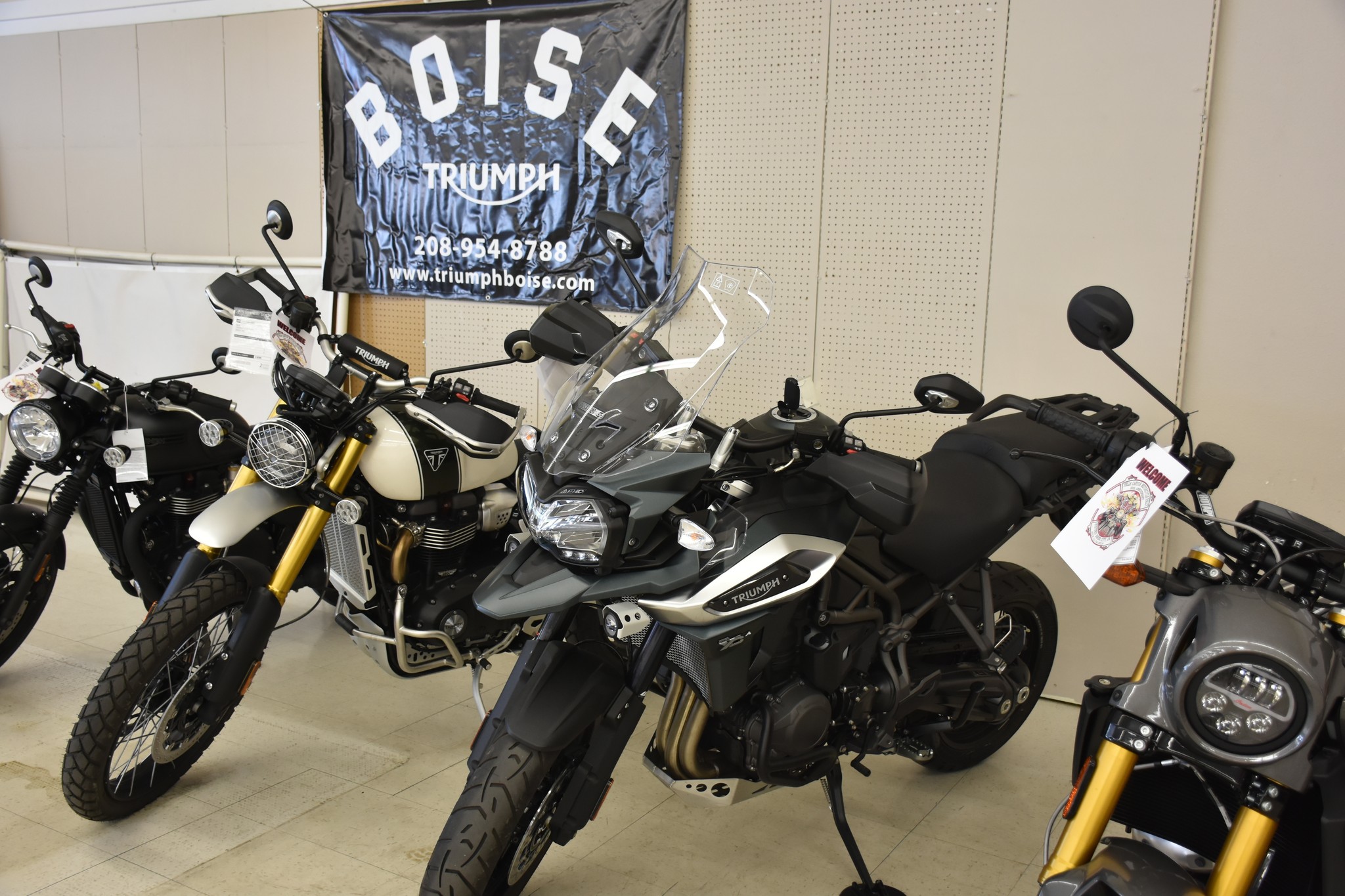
415 210 1157 896
56 200 626 825
1030 283 1345 896
1 254 339 671
